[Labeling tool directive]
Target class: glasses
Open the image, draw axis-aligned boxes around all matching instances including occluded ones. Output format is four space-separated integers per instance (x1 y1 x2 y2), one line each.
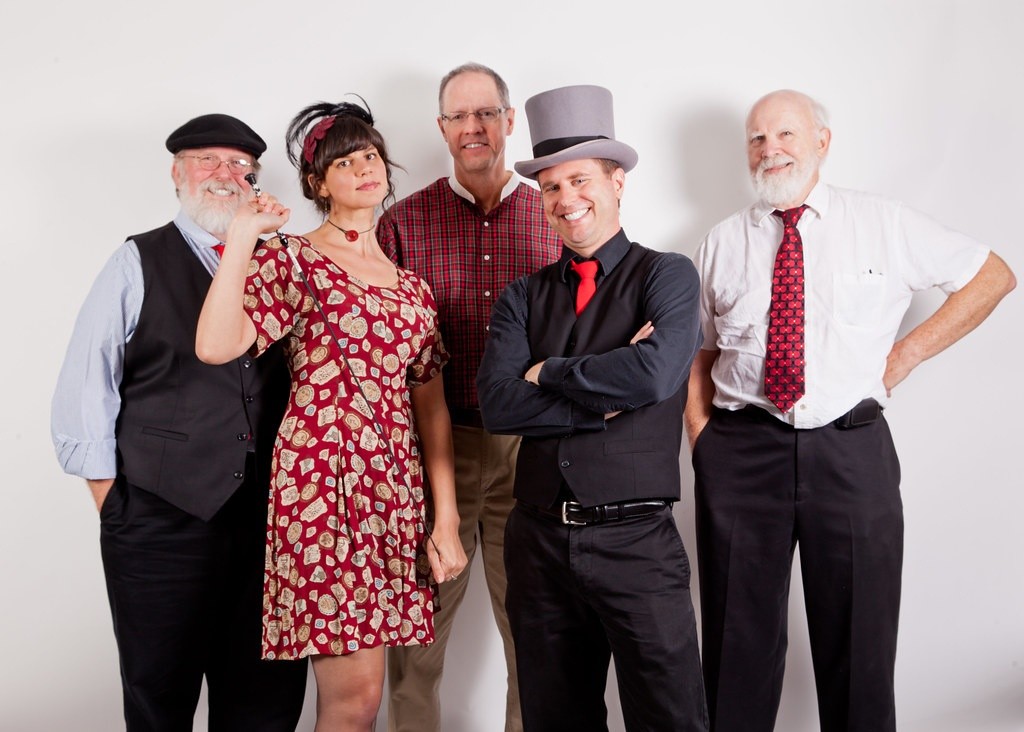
441 106 507 127
179 154 254 175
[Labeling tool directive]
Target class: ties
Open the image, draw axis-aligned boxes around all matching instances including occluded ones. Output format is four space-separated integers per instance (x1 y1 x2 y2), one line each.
764 204 810 414
210 244 225 259
568 258 600 319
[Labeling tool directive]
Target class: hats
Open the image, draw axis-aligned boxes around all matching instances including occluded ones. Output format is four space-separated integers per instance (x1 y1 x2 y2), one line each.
166 114 267 160
514 85 638 180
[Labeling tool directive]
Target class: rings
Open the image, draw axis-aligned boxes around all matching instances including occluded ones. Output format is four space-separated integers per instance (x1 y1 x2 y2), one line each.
451 573 458 581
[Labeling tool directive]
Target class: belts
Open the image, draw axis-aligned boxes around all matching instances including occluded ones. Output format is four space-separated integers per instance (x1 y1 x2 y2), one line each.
447 407 484 427
515 500 671 526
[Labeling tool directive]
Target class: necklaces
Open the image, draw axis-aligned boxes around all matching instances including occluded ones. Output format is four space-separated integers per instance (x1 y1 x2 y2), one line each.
325 218 375 244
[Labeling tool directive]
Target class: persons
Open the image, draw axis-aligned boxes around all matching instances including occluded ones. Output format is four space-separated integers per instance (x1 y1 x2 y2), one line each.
50 112 315 732
679 86 1018 732
195 100 470 732
472 84 712 732
372 59 566 732
